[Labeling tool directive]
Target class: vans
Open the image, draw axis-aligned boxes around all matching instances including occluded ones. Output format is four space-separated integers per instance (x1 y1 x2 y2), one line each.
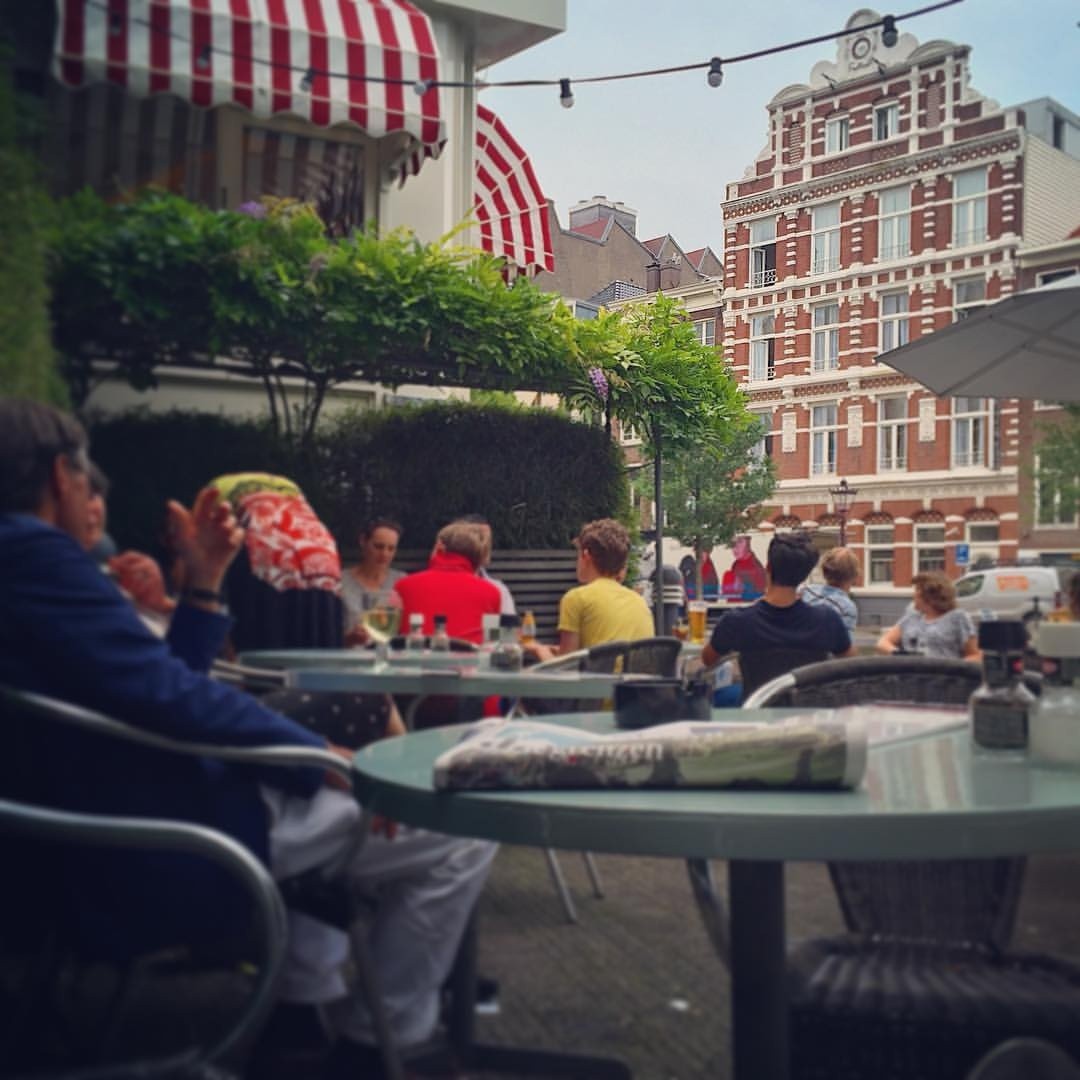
948 566 1063 625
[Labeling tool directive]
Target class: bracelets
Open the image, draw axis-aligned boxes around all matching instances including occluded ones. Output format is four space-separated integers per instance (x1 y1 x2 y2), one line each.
181 586 228 604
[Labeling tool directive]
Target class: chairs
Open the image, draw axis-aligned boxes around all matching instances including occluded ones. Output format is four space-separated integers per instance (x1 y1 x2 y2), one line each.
682 651 1080 1080
0 636 682 1080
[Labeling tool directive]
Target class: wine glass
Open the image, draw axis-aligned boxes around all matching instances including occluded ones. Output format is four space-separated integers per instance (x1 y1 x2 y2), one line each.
361 591 405 674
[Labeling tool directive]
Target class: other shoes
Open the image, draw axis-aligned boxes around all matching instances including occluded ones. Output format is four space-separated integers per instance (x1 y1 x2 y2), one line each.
267 975 501 1064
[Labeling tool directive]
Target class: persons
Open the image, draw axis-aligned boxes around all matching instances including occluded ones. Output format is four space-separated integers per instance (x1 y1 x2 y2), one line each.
793 546 860 659
1063 573 1080 616
875 568 984 664
0 397 503 1080
701 531 855 700
521 517 657 713
80 471 517 740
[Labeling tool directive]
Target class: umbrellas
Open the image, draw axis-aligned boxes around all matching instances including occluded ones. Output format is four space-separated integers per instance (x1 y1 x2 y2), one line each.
872 271 1080 403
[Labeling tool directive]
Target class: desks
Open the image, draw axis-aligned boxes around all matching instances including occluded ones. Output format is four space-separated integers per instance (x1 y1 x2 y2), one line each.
353 699 1080 1080
233 651 615 729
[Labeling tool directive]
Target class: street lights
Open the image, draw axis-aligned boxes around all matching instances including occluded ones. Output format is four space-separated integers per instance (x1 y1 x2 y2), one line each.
828 479 859 546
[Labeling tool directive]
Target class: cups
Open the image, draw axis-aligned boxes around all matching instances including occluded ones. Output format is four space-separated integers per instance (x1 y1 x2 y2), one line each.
688 600 709 645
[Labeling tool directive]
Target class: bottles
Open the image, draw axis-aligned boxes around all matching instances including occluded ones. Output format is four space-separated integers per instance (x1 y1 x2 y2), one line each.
489 614 524 671
521 609 537 645
404 613 425 648
968 620 1037 748
1025 620 1080 768
429 614 451 650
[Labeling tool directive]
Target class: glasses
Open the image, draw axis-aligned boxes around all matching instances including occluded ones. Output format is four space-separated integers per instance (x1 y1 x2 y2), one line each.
67 459 109 499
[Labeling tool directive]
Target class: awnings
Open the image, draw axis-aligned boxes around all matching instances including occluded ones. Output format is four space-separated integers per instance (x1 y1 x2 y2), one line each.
471 104 555 285
48 0 448 191
